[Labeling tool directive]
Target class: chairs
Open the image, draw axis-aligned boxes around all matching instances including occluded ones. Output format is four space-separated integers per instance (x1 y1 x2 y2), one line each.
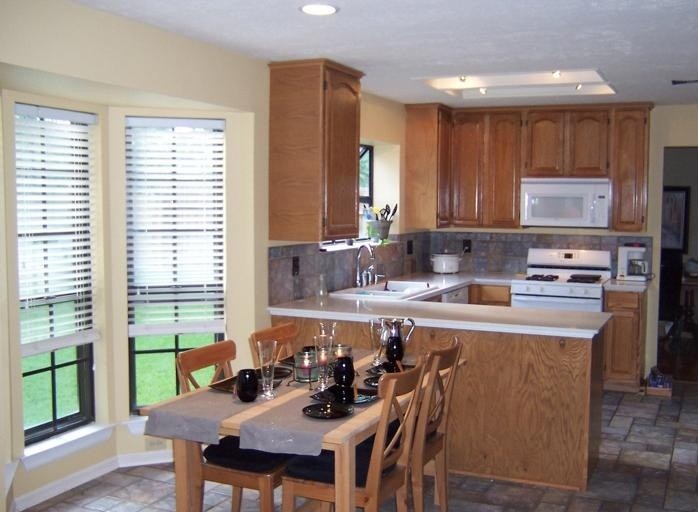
248 322 299 370
281 354 429 512
176 340 284 511
395 336 459 512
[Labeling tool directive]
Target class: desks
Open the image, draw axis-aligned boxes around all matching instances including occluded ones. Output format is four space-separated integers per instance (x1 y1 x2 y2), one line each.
680 280 698 325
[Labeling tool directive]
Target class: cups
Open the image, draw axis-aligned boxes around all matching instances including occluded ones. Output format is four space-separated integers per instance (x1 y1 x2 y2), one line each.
296 351 318 382
336 344 352 361
367 318 404 365
237 368 258 402
320 321 336 338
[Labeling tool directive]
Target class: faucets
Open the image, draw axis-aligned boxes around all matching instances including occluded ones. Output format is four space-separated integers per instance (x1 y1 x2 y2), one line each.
355 243 375 287
374 272 386 284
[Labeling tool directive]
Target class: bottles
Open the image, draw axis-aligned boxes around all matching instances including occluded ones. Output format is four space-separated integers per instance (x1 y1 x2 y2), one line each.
314 272 328 298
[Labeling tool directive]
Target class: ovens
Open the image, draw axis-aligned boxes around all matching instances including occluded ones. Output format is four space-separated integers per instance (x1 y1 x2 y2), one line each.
509 293 602 312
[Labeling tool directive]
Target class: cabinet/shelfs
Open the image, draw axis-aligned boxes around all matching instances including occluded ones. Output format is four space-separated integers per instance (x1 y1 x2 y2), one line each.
268 59 366 242
602 290 647 393
471 283 511 305
400 102 654 230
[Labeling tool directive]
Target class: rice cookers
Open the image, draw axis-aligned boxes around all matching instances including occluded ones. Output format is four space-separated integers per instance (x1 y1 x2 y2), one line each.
429 251 464 274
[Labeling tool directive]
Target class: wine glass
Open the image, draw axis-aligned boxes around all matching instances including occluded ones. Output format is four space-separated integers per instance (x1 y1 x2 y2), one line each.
313 334 332 391
256 338 278 400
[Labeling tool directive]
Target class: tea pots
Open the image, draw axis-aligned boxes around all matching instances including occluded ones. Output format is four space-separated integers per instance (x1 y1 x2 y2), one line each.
385 317 416 367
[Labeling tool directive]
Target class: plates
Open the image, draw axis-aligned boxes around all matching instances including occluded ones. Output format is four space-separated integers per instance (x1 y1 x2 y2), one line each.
364 375 383 388
365 362 414 375
281 354 296 364
255 368 292 380
312 384 379 404
212 374 282 400
303 403 356 419
301 344 314 353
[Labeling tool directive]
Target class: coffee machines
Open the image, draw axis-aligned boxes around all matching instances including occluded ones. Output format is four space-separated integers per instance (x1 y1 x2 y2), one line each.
616 242 655 282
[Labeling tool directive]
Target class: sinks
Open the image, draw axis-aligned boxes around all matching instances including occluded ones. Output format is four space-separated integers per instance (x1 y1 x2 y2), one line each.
361 279 440 294
329 287 416 300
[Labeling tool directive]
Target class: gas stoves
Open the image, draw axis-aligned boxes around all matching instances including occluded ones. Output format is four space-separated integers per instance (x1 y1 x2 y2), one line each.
510 268 613 298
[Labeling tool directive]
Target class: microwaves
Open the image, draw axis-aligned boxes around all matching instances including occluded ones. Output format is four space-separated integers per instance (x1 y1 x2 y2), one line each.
519 176 613 230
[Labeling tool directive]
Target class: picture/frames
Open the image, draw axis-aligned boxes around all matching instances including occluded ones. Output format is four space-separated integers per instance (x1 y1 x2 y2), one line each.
661 185 691 255
